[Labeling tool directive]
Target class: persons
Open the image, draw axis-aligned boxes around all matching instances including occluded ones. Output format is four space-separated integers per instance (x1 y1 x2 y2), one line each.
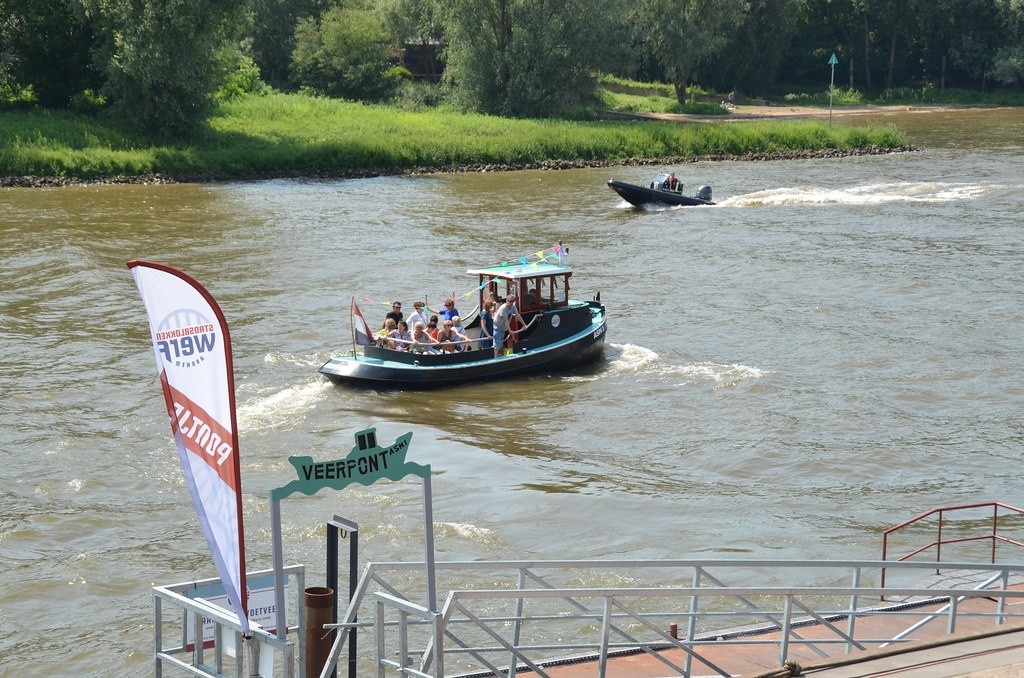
410 321 440 355
383 321 412 351
669 173 678 190
478 301 496 349
483 292 501 320
450 315 472 351
492 294 527 358
427 299 459 321
376 318 397 350
423 315 441 339
529 290 537 297
406 302 428 330
381 301 404 330
436 320 471 354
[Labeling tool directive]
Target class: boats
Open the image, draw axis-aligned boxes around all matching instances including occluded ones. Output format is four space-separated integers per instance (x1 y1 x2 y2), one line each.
317 240 607 392
607 171 717 207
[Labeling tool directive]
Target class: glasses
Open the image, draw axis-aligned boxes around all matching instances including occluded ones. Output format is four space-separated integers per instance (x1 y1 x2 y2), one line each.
393 306 401 309
446 325 451 326
444 304 451 307
507 300 514 303
